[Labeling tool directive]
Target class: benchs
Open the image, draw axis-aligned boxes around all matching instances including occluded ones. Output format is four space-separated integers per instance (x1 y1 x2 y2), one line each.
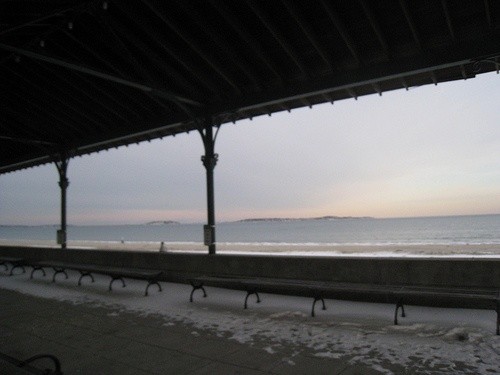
0 255 500 336
0 351 63 375
